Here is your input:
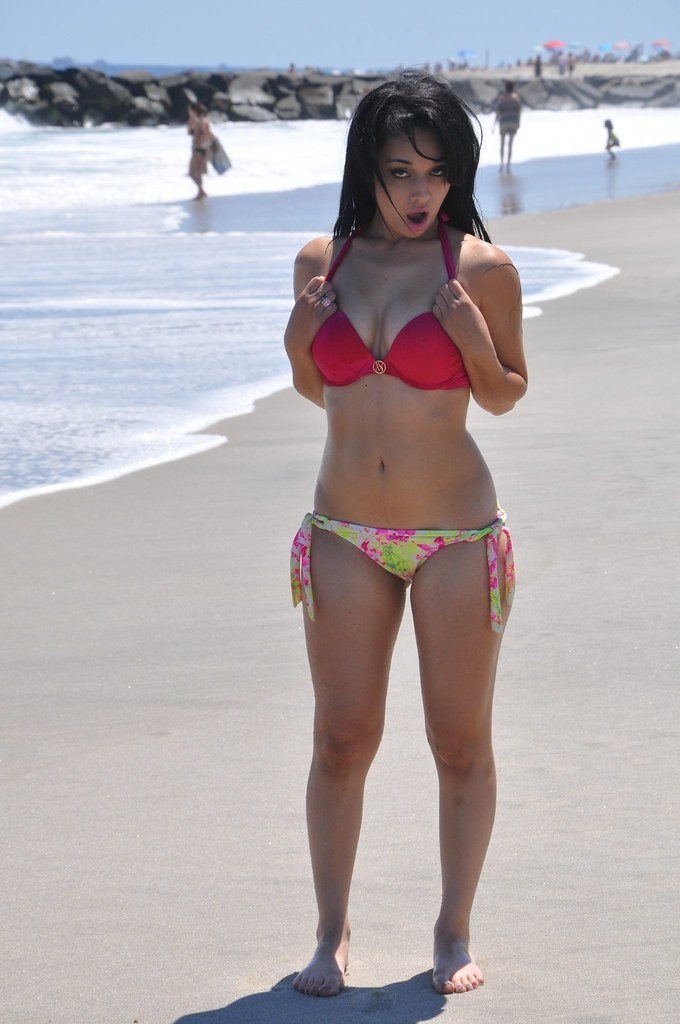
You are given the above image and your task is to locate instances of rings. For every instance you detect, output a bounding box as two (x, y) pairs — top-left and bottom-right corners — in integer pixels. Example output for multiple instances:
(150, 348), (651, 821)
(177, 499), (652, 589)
(320, 295), (332, 308)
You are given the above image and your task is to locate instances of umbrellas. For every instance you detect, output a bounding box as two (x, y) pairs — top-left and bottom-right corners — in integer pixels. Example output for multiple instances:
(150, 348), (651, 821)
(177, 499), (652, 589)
(459, 37), (672, 58)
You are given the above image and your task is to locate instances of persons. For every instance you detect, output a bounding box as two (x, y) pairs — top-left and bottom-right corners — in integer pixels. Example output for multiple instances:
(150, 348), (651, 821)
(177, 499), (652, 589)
(186, 102), (217, 200)
(281, 75), (530, 997)
(419, 49), (679, 76)
(604, 118), (621, 161)
(493, 80), (523, 171)
